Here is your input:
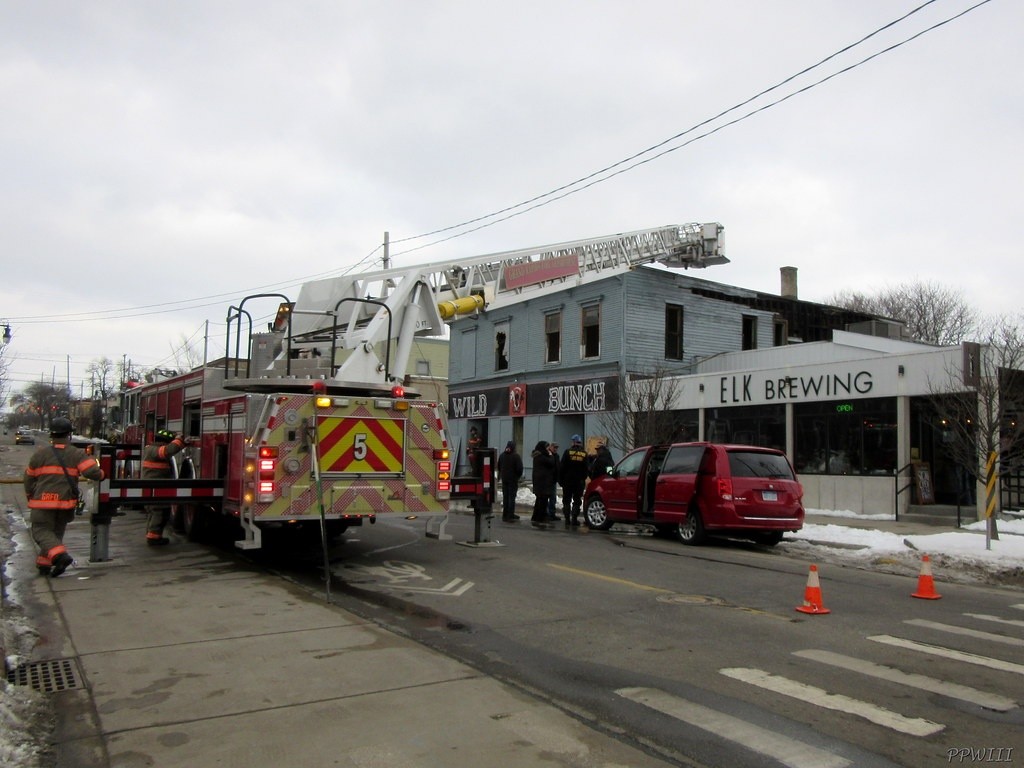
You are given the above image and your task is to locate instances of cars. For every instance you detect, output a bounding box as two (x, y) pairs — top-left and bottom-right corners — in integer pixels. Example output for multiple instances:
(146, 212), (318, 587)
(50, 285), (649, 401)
(15, 429), (35, 445)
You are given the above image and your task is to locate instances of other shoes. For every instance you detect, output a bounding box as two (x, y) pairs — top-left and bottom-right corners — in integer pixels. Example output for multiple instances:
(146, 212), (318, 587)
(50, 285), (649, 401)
(147, 537), (170, 546)
(51, 552), (73, 577)
(548, 514), (561, 521)
(502, 518), (515, 523)
(531, 517), (547, 522)
(512, 515), (520, 519)
(38, 565), (51, 575)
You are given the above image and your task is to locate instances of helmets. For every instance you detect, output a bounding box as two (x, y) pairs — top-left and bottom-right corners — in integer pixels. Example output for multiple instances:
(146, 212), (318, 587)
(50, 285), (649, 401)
(572, 434), (582, 443)
(50, 417), (77, 435)
(156, 430), (175, 442)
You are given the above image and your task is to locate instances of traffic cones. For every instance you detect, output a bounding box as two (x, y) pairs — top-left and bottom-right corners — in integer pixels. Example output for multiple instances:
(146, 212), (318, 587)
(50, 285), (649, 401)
(794, 561), (832, 614)
(910, 554), (942, 599)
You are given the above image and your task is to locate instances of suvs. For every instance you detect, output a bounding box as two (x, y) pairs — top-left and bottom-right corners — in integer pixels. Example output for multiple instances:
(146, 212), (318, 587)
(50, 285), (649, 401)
(583, 441), (805, 548)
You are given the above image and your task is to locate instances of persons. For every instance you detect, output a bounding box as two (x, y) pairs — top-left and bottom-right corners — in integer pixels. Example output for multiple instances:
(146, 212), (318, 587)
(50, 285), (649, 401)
(141, 429), (186, 546)
(109, 422), (123, 444)
(530, 440), (562, 528)
(24, 416), (106, 577)
(560, 435), (590, 527)
(589, 446), (615, 480)
(467, 426), (482, 476)
(497, 440), (523, 523)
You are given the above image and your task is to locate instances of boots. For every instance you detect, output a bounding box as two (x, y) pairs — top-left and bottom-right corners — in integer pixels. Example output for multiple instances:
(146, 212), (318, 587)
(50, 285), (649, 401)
(565, 514), (571, 525)
(571, 514), (580, 526)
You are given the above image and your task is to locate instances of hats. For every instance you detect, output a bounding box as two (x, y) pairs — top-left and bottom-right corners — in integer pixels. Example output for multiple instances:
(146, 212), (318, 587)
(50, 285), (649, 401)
(551, 442), (559, 447)
(470, 426), (478, 432)
(595, 444), (609, 451)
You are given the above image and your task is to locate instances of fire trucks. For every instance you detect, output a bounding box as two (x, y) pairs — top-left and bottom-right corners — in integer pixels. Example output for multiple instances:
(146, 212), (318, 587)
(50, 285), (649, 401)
(70, 220), (730, 571)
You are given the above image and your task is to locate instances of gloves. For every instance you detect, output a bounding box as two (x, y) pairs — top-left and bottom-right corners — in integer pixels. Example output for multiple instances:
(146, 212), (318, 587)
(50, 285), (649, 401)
(100, 468), (106, 482)
(176, 434), (185, 442)
